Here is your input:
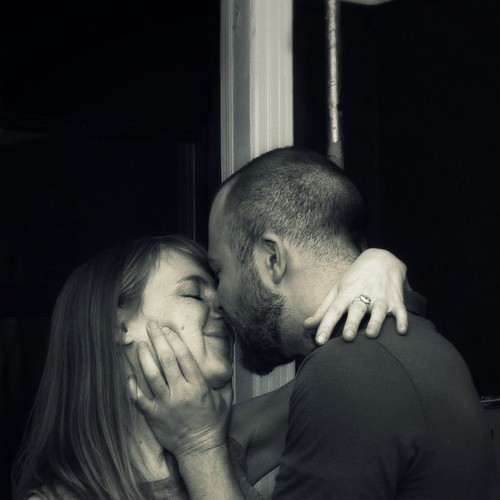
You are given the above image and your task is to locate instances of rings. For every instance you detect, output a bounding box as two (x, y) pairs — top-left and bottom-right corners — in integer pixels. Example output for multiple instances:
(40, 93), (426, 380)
(352, 293), (371, 311)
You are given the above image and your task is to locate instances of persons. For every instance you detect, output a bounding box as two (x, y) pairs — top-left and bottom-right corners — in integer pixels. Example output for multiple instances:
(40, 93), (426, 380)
(128, 146), (500, 500)
(11, 233), (412, 500)
